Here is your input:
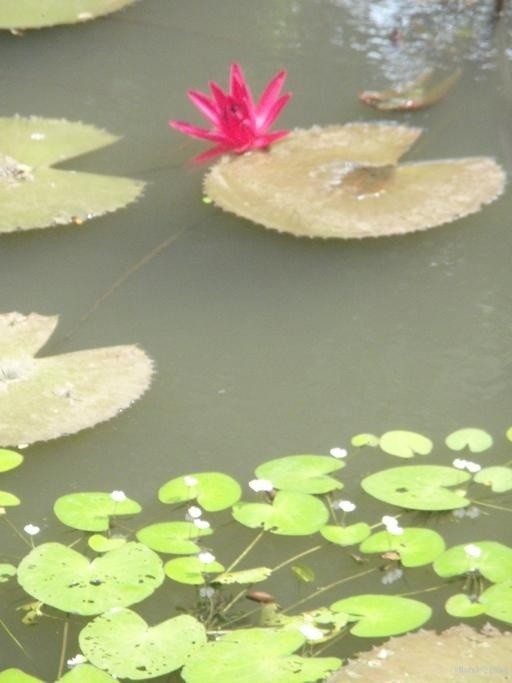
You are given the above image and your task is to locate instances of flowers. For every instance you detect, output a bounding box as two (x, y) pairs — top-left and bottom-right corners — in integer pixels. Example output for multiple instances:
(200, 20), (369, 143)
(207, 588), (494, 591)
(168, 64), (293, 166)
